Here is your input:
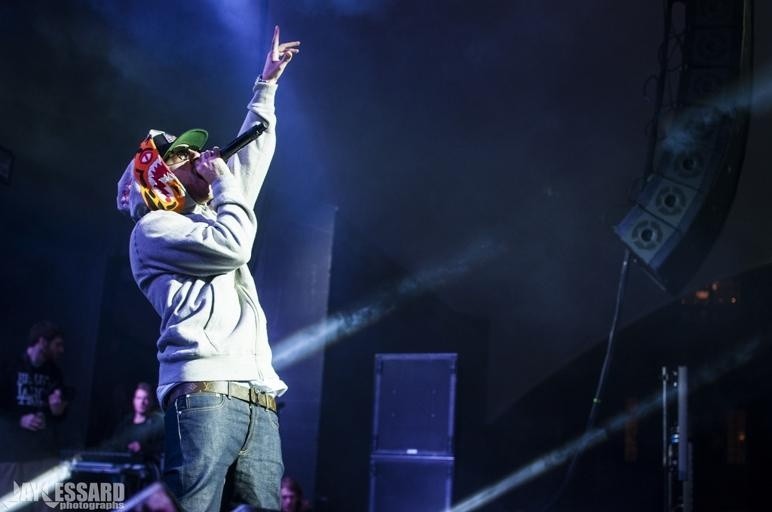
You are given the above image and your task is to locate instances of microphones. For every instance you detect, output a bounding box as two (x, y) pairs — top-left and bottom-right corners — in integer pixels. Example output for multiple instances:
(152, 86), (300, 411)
(192, 121), (269, 165)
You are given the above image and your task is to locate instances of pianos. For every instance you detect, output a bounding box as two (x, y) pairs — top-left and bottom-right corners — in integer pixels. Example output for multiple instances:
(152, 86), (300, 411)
(70, 451), (139, 465)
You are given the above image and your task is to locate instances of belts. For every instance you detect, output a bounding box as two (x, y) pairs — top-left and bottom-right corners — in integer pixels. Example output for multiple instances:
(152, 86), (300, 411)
(163, 381), (277, 413)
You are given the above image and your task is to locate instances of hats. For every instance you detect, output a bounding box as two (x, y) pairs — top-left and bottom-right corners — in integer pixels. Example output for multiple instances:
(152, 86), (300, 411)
(154, 128), (208, 163)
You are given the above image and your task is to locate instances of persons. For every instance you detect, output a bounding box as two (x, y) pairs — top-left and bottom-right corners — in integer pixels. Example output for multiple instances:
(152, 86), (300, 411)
(0, 318), (71, 462)
(110, 23), (303, 512)
(111, 380), (163, 492)
(281, 477), (311, 512)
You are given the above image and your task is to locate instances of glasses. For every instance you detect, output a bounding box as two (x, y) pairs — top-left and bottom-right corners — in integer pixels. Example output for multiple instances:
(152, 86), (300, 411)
(174, 146), (200, 160)
